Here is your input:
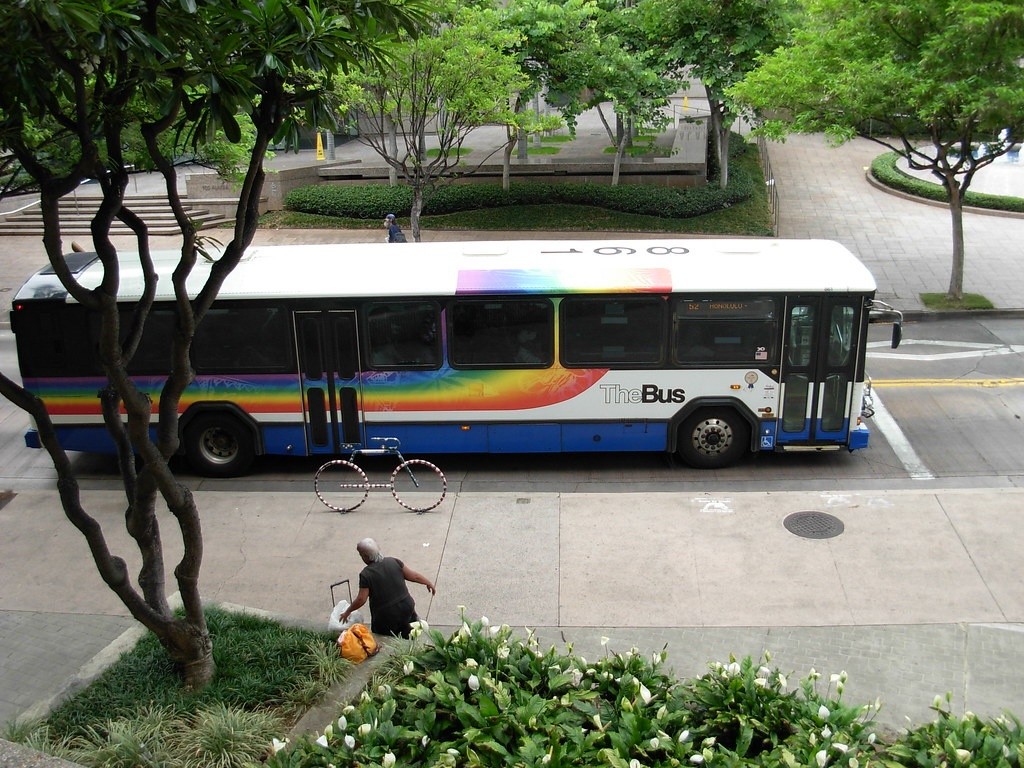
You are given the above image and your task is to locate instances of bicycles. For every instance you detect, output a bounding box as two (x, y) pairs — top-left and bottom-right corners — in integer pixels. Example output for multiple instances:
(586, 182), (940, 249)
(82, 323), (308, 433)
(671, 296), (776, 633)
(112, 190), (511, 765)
(313, 436), (448, 515)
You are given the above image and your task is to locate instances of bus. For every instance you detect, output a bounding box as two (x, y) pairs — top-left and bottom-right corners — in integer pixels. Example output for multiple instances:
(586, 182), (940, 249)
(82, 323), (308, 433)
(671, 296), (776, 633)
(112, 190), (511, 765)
(8, 238), (905, 479)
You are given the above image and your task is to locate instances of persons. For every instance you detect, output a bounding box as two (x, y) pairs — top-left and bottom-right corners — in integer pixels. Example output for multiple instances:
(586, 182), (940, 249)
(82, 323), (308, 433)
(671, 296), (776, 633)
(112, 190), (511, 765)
(339, 538), (436, 640)
(384, 214), (408, 243)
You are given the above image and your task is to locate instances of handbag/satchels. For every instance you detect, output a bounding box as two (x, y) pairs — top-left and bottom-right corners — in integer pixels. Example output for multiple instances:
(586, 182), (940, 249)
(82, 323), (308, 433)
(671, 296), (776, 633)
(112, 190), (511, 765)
(341, 623), (381, 663)
(329, 600), (364, 632)
